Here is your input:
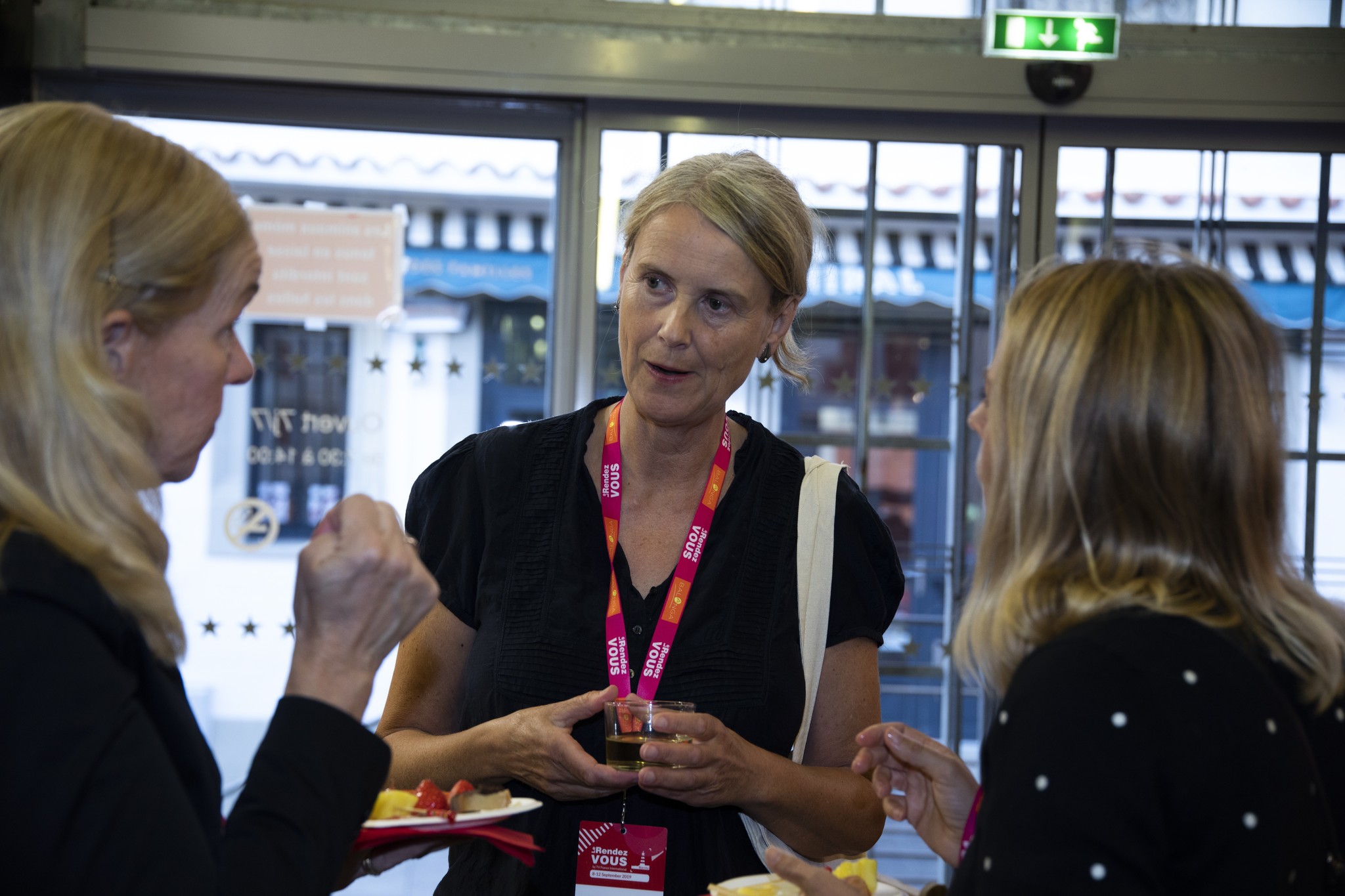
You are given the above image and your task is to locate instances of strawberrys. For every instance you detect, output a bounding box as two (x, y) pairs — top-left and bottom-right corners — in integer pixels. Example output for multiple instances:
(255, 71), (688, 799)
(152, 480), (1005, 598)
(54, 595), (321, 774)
(446, 779), (474, 805)
(412, 780), (449, 816)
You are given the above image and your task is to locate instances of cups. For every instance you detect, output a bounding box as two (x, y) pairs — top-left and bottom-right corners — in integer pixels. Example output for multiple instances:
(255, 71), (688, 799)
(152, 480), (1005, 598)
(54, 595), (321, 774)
(602, 697), (694, 772)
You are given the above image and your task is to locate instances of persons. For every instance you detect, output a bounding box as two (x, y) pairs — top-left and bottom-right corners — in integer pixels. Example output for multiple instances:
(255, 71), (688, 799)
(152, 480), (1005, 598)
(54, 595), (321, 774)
(375, 149), (905, 896)
(1, 102), (441, 896)
(762, 240), (1345, 896)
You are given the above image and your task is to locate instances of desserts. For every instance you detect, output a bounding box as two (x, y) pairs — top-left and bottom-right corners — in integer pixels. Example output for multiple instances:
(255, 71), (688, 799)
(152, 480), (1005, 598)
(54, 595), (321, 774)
(707, 857), (878, 896)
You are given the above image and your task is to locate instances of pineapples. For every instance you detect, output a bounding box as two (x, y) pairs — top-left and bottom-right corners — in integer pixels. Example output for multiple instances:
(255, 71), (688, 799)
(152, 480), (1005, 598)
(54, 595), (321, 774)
(369, 789), (418, 819)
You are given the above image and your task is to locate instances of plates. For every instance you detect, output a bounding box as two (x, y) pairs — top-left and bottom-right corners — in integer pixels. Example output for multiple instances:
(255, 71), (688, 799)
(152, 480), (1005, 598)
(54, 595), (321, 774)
(361, 796), (542, 828)
(709, 873), (905, 896)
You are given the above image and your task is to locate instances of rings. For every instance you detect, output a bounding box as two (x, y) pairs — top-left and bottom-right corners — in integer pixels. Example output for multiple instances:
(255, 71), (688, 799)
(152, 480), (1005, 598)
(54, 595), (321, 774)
(404, 535), (418, 550)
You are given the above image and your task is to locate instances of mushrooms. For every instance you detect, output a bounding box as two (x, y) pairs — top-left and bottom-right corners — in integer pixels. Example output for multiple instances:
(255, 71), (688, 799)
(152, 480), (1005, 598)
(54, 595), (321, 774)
(451, 789), (511, 812)
